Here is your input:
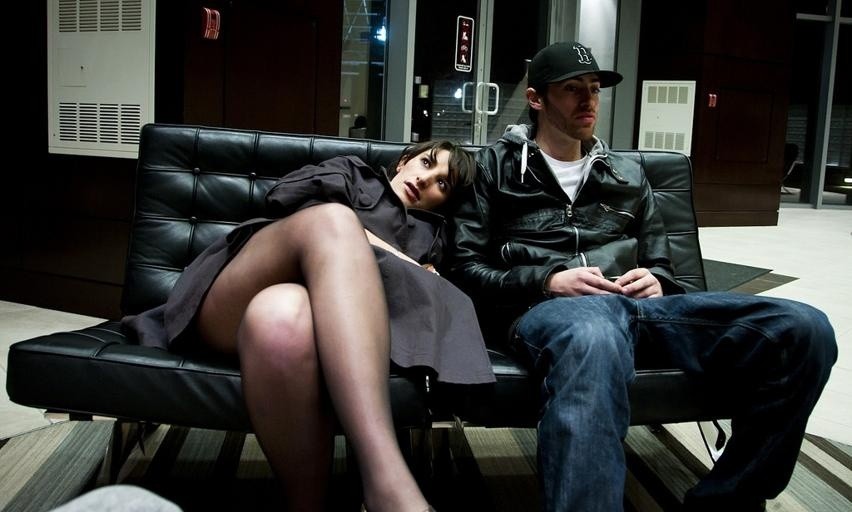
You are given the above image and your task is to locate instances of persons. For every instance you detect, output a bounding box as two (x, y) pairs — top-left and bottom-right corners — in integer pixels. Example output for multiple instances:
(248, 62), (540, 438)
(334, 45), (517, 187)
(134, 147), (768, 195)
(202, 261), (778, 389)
(454, 42), (838, 512)
(122, 138), (498, 511)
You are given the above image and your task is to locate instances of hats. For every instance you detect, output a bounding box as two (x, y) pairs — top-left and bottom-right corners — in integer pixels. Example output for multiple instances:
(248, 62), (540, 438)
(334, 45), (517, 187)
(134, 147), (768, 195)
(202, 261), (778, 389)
(528, 41), (623, 88)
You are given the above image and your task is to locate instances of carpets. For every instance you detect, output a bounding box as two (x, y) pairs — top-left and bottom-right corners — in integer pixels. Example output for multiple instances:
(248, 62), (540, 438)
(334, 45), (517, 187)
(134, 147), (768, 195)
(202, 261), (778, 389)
(1, 421), (852, 512)
(702, 259), (801, 295)
